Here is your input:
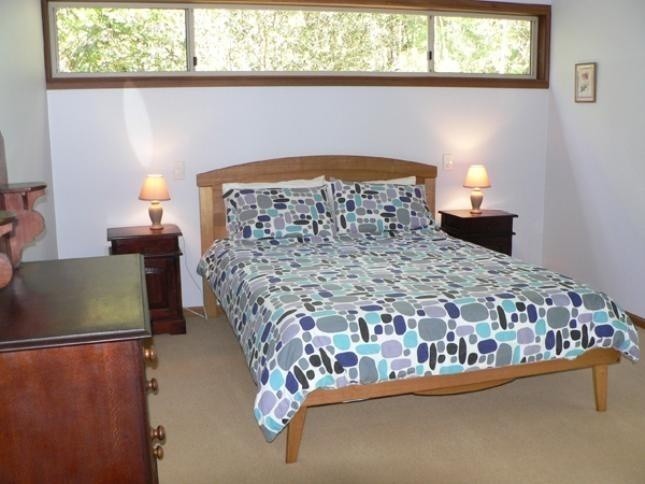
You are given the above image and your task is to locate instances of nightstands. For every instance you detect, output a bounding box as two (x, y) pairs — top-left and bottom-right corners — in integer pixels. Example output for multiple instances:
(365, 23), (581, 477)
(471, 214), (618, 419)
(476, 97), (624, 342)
(438, 208), (519, 258)
(104, 222), (191, 337)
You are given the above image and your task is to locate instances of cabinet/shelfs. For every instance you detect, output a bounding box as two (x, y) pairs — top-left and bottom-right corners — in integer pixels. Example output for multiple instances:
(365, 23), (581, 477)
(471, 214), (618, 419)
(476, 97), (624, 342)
(0, 250), (166, 483)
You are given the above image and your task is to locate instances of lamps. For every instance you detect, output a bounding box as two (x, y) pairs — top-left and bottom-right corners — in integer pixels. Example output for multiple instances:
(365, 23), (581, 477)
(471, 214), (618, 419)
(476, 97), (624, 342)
(135, 171), (174, 230)
(463, 162), (491, 215)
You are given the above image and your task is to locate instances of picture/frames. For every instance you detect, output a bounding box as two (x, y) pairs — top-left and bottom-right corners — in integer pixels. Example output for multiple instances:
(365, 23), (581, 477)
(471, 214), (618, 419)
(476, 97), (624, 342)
(574, 59), (597, 103)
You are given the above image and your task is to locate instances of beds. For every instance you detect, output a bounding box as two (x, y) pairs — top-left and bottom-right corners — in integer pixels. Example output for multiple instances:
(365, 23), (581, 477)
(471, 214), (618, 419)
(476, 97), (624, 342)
(193, 150), (641, 465)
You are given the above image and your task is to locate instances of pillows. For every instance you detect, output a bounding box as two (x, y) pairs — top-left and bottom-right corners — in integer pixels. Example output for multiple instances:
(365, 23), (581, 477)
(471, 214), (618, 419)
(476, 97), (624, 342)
(329, 173), (417, 185)
(219, 170), (327, 191)
(332, 178), (436, 234)
(219, 184), (336, 246)
